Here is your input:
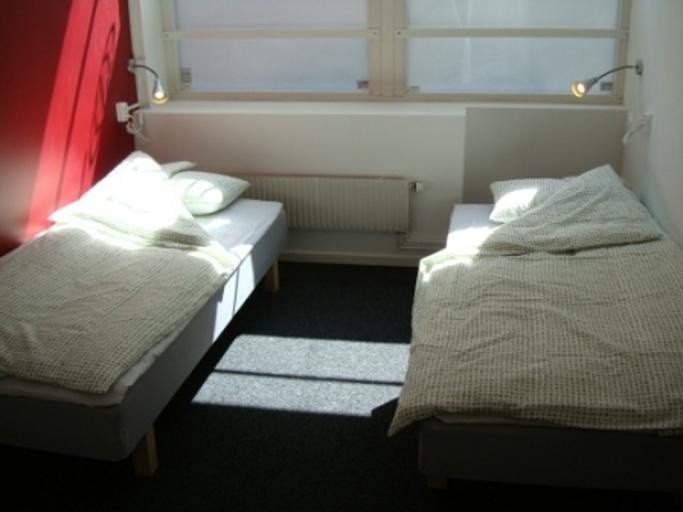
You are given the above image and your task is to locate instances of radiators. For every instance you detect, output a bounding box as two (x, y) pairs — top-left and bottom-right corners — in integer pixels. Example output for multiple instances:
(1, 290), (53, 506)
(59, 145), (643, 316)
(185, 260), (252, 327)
(231, 170), (411, 236)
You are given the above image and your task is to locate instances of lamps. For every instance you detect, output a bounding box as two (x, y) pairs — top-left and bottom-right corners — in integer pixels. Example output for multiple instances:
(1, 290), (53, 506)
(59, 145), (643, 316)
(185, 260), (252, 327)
(128, 59), (169, 105)
(570, 59), (643, 99)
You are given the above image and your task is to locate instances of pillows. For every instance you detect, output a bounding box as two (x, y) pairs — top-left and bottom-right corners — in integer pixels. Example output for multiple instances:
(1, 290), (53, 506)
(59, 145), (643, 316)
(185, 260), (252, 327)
(154, 157), (253, 216)
(487, 176), (581, 224)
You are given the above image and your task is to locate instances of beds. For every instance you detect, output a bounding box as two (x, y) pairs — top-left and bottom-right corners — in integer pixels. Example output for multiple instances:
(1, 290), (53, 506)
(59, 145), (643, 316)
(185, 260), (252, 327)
(417, 199), (680, 499)
(2, 191), (295, 481)
